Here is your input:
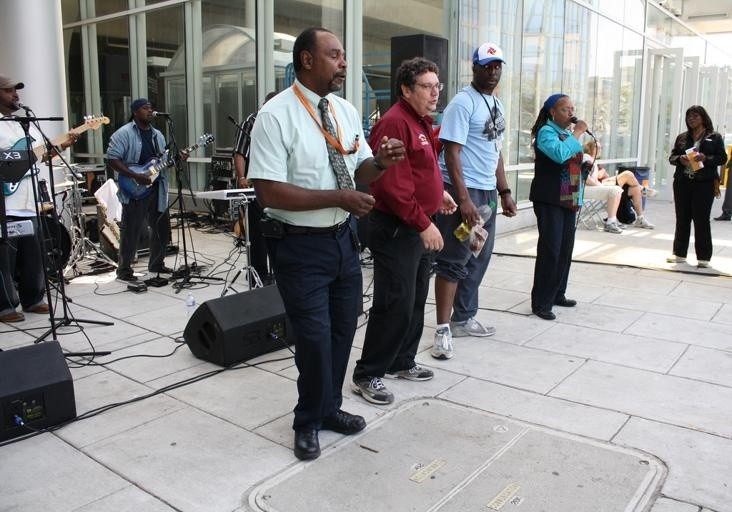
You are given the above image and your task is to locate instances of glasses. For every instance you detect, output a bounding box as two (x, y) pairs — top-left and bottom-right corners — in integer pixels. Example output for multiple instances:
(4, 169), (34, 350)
(416, 82), (444, 91)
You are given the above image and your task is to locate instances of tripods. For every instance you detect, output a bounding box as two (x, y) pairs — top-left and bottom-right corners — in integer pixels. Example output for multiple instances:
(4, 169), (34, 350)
(0, 116), (115, 358)
(164, 124), (224, 295)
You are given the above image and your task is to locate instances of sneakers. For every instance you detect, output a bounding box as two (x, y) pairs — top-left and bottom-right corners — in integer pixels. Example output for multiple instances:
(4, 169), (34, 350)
(714, 214), (731, 220)
(450, 317), (496, 338)
(697, 260), (708, 267)
(634, 218), (654, 229)
(640, 186), (657, 198)
(350, 377), (394, 404)
(603, 222), (622, 234)
(667, 255), (685, 263)
(432, 327), (453, 360)
(384, 364), (433, 381)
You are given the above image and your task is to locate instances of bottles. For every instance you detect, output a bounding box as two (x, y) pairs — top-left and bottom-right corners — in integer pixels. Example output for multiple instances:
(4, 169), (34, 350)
(454, 201), (496, 242)
(186, 291), (197, 322)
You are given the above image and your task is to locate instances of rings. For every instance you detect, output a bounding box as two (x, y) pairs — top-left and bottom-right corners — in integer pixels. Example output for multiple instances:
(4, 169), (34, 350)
(74, 139), (78, 142)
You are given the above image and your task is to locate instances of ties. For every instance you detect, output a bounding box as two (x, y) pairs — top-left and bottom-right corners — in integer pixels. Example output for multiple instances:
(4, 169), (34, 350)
(318, 98), (354, 189)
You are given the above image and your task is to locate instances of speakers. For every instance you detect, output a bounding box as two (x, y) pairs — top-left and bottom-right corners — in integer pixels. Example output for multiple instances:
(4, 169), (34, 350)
(0, 339), (77, 447)
(182, 283), (296, 368)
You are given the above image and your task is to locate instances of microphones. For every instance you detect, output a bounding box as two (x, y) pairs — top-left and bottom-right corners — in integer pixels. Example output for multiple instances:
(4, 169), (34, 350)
(570, 115), (592, 137)
(151, 109), (171, 121)
(226, 113), (250, 137)
(13, 101), (33, 112)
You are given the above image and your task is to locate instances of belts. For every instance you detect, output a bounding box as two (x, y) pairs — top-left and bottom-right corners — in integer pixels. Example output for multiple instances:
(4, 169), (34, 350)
(282, 220), (348, 233)
(678, 173), (702, 180)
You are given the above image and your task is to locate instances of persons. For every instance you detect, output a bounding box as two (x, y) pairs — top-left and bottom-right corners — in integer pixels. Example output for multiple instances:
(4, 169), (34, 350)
(104, 97), (192, 281)
(712, 154), (732, 222)
(429, 35), (519, 364)
(527, 93), (601, 322)
(582, 139), (627, 235)
(664, 105), (728, 269)
(245, 25), (409, 462)
(346, 52), (460, 407)
(0, 73), (82, 322)
(232, 89), (277, 289)
(581, 163), (653, 234)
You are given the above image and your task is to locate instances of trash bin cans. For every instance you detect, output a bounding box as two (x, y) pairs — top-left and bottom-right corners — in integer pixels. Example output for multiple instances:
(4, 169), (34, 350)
(617, 166), (651, 211)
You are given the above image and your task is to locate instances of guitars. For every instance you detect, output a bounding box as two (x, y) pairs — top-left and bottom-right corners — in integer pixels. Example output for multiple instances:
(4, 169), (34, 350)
(3, 113), (110, 197)
(119, 134), (215, 201)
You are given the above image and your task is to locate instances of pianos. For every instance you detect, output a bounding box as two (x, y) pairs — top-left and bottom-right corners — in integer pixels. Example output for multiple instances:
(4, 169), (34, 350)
(196, 185), (255, 200)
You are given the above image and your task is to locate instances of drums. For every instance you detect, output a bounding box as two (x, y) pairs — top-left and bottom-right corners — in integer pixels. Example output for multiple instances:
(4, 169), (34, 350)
(37, 200), (74, 270)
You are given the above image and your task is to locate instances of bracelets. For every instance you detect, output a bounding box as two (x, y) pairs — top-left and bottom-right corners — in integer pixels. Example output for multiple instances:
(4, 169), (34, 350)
(373, 156), (387, 172)
(56, 144), (63, 152)
(239, 176), (245, 180)
(497, 187), (511, 196)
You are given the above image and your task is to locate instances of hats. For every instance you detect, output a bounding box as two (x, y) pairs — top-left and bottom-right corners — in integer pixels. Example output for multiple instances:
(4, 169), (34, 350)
(128, 98), (151, 122)
(473, 43), (506, 66)
(0, 74), (24, 89)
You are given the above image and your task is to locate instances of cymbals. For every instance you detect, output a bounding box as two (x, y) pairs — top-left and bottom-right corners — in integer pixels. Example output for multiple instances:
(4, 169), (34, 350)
(53, 180), (85, 187)
(46, 163), (77, 168)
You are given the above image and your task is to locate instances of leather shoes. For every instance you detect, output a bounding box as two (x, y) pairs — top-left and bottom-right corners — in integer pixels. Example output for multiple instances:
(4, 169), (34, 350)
(0, 313), (25, 322)
(149, 266), (173, 273)
(532, 310), (556, 320)
(555, 300), (576, 306)
(116, 274), (137, 281)
(321, 409), (366, 434)
(294, 429), (321, 460)
(23, 304), (49, 313)
